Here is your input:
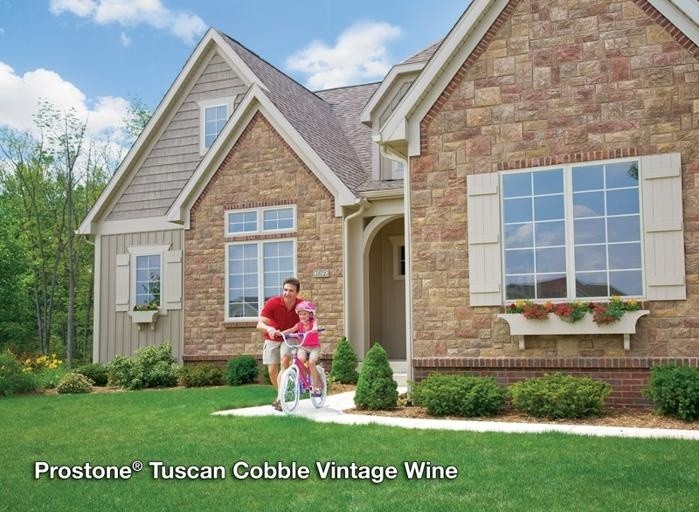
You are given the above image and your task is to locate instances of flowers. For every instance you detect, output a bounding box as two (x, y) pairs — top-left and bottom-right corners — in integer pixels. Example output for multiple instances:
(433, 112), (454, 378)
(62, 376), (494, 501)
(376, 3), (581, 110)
(507, 295), (640, 326)
(133, 303), (158, 311)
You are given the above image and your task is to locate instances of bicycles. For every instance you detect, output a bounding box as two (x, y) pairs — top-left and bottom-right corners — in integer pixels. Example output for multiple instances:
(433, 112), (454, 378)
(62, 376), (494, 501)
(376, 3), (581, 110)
(265, 327), (330, 415)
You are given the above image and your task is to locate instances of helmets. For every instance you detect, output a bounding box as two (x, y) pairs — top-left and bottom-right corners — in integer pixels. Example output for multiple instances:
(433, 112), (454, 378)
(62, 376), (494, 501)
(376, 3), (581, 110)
(295, 301), (316, 316)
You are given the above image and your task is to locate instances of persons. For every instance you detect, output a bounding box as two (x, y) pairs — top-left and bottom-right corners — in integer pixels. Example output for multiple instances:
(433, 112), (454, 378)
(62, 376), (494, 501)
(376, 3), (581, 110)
(275, 301), (322, 393)
(256, 278), (304, 412)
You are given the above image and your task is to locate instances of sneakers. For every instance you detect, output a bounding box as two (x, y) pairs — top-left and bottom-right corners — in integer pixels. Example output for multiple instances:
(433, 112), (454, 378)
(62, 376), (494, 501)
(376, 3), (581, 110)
(311, 382), (325, 397)
(272, 397), (283, 411)
